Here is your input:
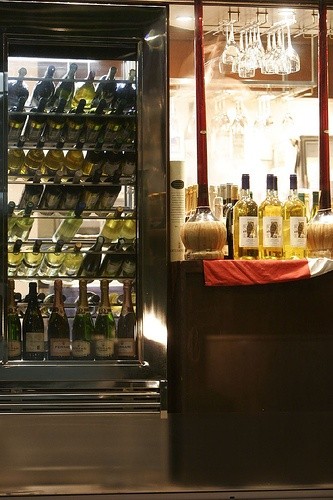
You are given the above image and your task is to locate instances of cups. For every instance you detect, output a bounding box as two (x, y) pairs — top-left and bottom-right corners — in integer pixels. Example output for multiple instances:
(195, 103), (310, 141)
(169, 159), (187, 262)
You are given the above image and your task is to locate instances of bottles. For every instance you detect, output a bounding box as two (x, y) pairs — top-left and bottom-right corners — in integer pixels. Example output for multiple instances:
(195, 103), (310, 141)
(311, 192), (319, 219)
(186, 171), (286, 257)
(5, 63), (138, 361)
(285, 174), (307, 256)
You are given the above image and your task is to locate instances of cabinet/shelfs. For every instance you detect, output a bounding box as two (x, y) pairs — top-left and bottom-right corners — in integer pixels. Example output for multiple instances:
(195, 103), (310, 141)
(2, 1), (168, 381)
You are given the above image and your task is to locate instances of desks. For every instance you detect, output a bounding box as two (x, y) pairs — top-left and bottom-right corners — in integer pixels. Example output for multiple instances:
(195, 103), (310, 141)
(168, 256), (333, 500)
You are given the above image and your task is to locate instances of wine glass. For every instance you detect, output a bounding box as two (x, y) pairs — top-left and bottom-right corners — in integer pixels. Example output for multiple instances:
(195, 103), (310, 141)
(220, 19), (301, 78)
(213, 84), (298, 139)
